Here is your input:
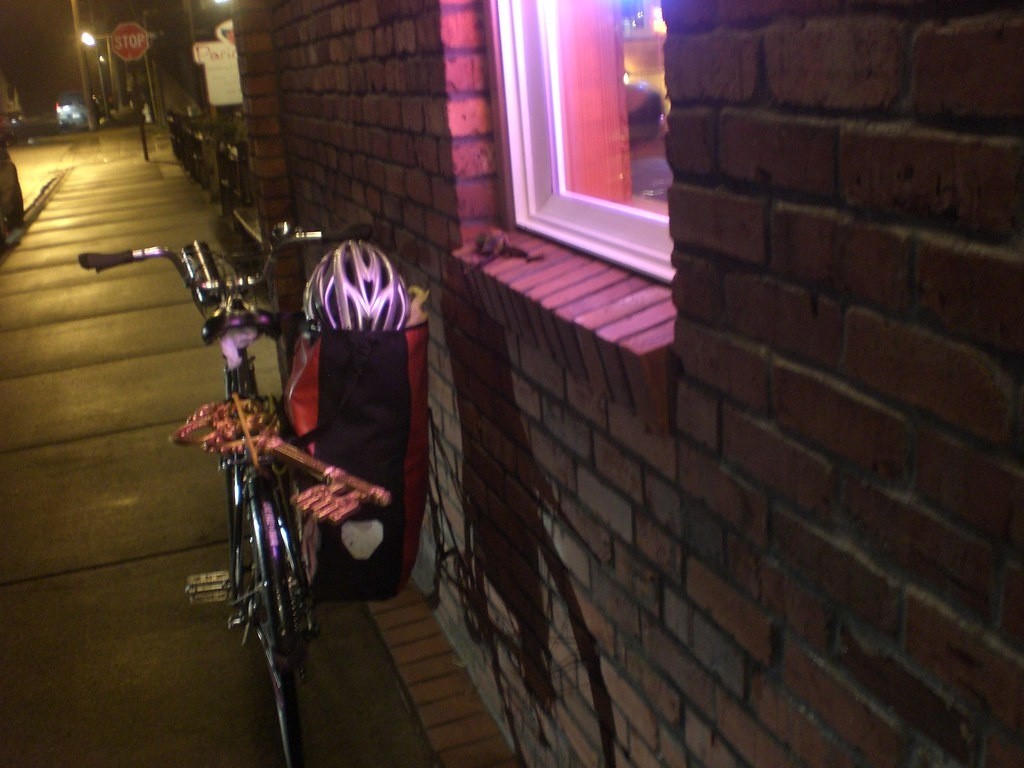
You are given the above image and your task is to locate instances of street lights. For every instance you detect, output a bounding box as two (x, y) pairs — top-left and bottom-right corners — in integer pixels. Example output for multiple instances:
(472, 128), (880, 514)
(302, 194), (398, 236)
(81, 31), (125, 117)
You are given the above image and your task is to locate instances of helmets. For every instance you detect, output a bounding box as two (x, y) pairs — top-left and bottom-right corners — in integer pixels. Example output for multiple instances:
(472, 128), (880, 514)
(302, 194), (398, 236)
(302, 239), (409, 332)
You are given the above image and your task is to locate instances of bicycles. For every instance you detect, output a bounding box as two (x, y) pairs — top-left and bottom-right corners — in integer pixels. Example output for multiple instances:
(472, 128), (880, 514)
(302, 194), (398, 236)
(76, 219), (376, 768)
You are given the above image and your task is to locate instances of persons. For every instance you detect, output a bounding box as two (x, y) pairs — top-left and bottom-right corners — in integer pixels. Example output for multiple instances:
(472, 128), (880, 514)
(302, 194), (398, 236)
(0, 138), (24, 242)
(625, 83), (674, 202)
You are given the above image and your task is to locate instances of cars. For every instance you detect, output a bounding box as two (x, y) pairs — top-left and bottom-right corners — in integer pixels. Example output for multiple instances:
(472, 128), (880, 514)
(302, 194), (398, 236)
(55, 92), (100, 130)
(0, 139), (25, 244)
(0, 109), (27, 146)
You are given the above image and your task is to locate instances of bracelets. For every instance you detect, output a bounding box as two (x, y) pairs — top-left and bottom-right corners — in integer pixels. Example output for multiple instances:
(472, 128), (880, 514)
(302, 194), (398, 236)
(3, 216), (8, 221)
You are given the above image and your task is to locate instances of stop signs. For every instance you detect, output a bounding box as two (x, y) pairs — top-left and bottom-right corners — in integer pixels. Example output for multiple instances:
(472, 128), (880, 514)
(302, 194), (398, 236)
(111, 21), (150, 61)
